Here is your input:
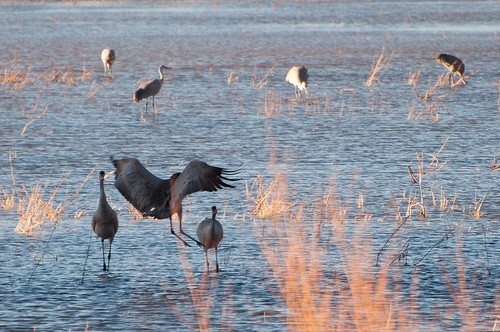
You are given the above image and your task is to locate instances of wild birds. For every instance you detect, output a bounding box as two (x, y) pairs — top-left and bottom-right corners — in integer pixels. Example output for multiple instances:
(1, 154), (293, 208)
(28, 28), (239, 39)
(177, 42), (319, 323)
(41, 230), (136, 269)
(434, 54), (467, 85)
(101, 48), (116, 73)
(133, 64), (172, 113)
(111, 158), (248, 248)
(285, 64), (309, 95)
(91, 171), (118, 271)
(197, 206), (223, 274)
